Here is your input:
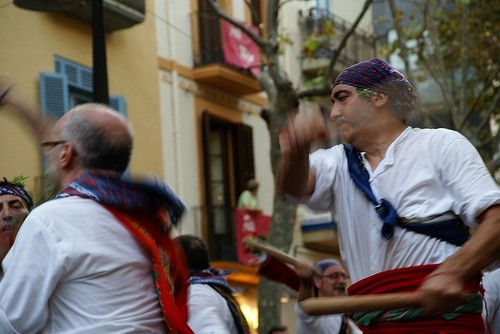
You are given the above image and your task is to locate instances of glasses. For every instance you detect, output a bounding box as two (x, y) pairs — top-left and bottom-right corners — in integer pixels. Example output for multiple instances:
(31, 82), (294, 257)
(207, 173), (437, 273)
(319, 272), (348, 280)
(41, 140), (79, 158)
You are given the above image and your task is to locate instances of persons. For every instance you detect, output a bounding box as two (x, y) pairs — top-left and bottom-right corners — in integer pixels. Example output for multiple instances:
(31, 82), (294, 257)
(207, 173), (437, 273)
(236, 179), (262, 210)
(0, 178), (34, 279)
(173, 235), (250, 334)
(481, 267), (500, 334)
(0, 102), (195, 334)
(276, 59), (500, 333)
(268, 258), (363, 334)
